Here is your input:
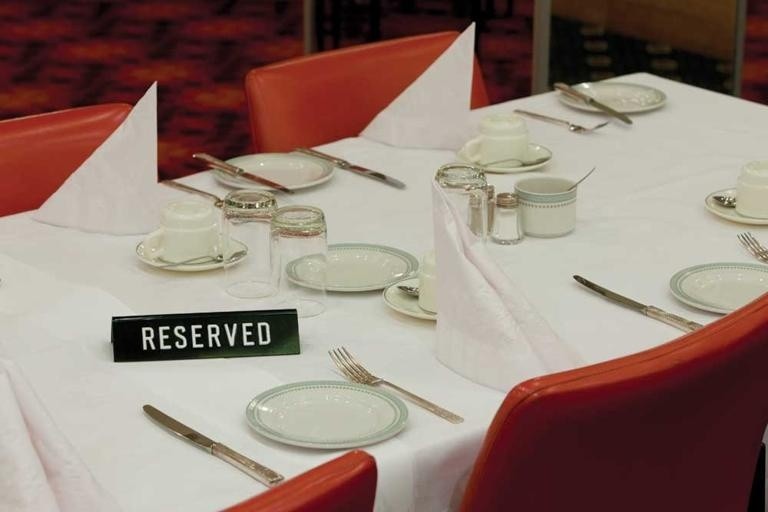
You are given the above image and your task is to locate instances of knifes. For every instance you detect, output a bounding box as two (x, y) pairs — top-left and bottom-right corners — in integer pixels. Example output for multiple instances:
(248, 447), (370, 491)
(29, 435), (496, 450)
(138, 401), (290, 488)
(568, 273), (706, 334)
(394, 279), (422, 300)
(294, 143), (410, 191)
(552, 80), (633, 126)
(194, 148), (296, 199)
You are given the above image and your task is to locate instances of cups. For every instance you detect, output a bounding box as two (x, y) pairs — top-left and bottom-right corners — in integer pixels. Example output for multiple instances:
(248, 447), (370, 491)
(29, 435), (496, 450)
(142, 200), (223, 260)
(514, 175), (576, 238)
(436, 163), (487, 249)
(477, 113), (531, 166)
(417, 251), (439, 313)
(733, 160), (768, 218)
(265, 206), (333, 320)
(220, 190), (282, 297)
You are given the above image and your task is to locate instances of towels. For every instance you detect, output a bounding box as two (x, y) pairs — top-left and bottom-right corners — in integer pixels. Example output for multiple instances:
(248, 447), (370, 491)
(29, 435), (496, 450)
(426, 176), (590, 390)
(31, 77), (168, 236)
(356, 20), (481, 154)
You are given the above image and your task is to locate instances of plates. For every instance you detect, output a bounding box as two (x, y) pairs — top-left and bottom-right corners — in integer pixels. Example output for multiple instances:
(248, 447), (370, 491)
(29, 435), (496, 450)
(458, 135), (555, 171)
(382, 279), (438, 324)
(667, 262), (768, 314)
(210, 152), (332, 191)
(288, 241), (420, 294)
(245, 378), (407, 452)
(705, 186), (768, 225)
(560, 81), (667, 115)
(135, 230), (248, 272)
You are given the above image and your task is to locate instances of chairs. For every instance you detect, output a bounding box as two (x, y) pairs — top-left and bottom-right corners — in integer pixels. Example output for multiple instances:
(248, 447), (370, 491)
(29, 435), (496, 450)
(223, 449), (380, 512)
(455, 289), (766, 509)
(0, 103), (138, 221)
(241, 29), (491, 152)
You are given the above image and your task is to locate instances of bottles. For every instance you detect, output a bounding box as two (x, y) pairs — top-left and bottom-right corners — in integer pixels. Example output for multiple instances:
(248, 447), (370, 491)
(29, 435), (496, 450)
(471, 180), (522, 247)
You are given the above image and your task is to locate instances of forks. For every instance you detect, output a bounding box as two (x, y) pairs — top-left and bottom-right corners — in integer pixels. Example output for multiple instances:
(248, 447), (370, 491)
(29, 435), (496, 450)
(514, 106), (609, 134)
(737, 230), (768, 266)
(328, 345), (468, 427)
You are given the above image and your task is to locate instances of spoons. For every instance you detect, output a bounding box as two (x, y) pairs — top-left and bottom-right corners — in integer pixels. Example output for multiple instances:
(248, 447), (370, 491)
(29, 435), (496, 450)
(712, 192), (735, 209)
